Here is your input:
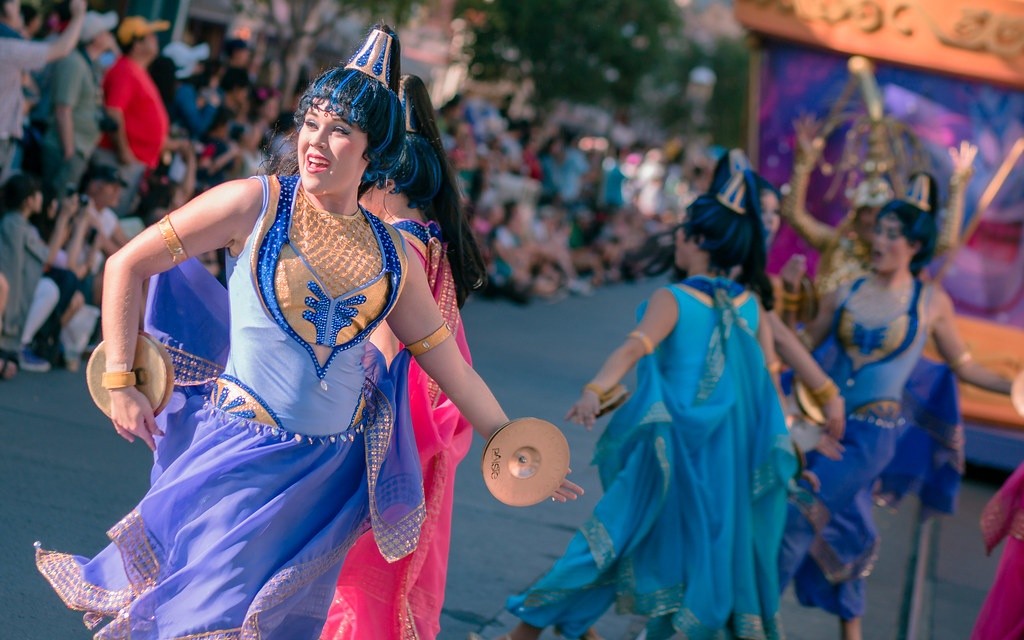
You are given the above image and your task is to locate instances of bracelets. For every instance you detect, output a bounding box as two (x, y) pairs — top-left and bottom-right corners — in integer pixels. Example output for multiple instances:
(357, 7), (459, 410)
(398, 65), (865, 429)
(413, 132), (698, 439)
(470, 190), (800, 640)
(582, 384), (605, 401)
(101, 372), (137, 390)
(812, 379), (838, 405)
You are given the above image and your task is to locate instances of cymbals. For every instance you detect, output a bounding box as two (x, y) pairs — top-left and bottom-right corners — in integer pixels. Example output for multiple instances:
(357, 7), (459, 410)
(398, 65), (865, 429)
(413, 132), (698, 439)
(481, 415), (575, 507)
(85, 328), (176, 423)
(788, 372), (837, 428)
(574, 382), (633, 422)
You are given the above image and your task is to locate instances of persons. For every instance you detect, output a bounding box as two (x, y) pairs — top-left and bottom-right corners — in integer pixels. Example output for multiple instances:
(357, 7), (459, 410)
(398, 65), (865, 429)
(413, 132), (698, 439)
(97, 64), (586, 639)
(430, 91), (729, 307)
(778, 199), (1016, 640)
(320, 70), (493, 640)
(785, 111), (978, 311)
(0, 1), (333, 381)
(972, 366), (1024, 640)
(747, 172), (849, 440)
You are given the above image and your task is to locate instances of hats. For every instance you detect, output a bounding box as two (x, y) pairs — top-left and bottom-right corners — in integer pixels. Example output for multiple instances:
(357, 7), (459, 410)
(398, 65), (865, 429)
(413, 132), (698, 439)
(76, 9), (117, 44)
(79, 164), (131, 193)
(161, 41), (211, 79)
(1, 173), (42, 210)
(117, 16), (170, 42)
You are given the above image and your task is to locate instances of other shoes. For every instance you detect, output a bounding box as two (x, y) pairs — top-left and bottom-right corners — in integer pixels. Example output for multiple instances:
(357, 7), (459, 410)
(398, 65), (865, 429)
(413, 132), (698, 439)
(18, 343), (50, 372)
